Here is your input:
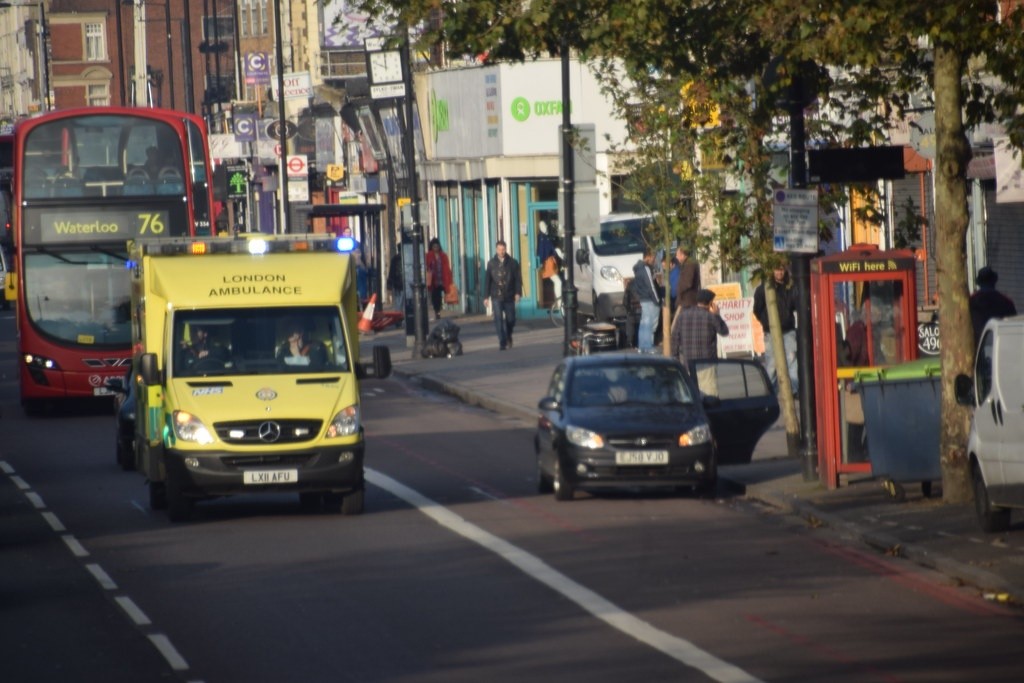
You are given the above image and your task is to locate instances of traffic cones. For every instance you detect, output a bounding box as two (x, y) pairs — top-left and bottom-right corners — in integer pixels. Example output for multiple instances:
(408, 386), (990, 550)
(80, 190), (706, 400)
(357, 293), (377, 335)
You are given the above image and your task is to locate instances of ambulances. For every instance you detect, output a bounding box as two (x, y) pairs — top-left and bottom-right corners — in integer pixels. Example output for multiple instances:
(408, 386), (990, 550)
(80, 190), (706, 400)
(124, 233), (394, 522)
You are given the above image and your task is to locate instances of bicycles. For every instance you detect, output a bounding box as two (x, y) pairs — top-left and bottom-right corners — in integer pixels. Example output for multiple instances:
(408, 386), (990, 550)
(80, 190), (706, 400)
(550, 295), (565, 329)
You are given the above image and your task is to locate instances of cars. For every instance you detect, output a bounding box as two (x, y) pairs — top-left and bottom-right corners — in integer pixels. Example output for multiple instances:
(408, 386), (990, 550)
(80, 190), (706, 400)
(533, 355), (781, 501)
(103, 365), (139, 470)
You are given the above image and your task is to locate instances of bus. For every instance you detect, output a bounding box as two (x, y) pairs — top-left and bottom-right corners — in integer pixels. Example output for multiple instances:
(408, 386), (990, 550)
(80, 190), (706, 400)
(0, 108), (216, 418)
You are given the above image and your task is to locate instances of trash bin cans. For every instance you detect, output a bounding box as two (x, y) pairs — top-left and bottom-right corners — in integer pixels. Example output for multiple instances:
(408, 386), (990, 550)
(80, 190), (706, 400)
(578, 324), (618, 357)
(851, 356), (941, 498)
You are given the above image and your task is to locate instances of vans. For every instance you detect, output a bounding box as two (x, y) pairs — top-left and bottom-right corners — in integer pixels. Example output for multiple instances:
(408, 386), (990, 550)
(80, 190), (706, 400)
(571, 211), (679, 349)
(952, 313), (1024, 533)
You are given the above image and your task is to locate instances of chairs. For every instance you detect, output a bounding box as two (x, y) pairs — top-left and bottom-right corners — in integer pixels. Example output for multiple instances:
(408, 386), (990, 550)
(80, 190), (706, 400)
(24, 167), (51, 198)
(156, 167), (185, 195)
(122, 167), (155, 196)
(573, 376), (607, 406)
(50, 167), (85, 197)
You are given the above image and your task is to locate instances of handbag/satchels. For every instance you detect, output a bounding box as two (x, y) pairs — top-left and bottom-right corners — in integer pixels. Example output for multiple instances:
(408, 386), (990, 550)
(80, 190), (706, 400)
(444, 283), (459, 305)
(425, 271), (433, 286)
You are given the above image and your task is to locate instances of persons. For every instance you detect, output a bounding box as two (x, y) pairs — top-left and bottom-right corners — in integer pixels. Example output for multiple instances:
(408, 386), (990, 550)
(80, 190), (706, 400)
(179, 325), (238, 376)
(623, 246), (902, 400)
(538, 221), (564, 311)
(328, 226), (523, 351)
(969, 267), (1016, 395)
(274, 315), (328, 367)
